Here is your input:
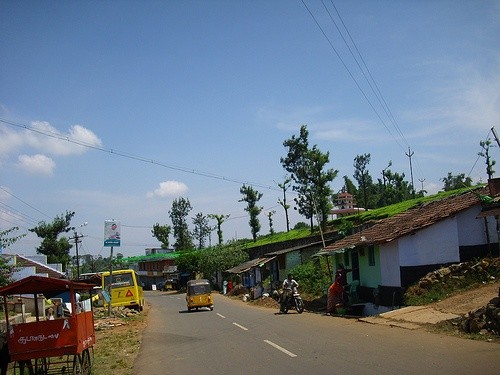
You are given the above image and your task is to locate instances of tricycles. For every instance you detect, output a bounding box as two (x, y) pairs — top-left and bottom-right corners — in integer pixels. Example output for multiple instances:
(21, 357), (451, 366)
(186, 279), (214, 311)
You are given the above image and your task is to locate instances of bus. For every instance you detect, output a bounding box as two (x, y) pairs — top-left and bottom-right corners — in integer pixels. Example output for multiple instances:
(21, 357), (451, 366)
(102, 270), (145, 312)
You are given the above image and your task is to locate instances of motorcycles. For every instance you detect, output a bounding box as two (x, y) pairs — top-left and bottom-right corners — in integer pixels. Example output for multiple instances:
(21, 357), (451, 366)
(279, 287), (305, 314)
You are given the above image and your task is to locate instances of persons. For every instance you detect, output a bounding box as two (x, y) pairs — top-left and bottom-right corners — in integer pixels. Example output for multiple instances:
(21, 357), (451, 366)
(105, 223), (120, 241)
(327, 274), (345, 316)
(279, 273), (299, 314)
(55, 300), (66, 317)
(76, 293), (80, 302)
(223, 278), (232, 296)
(480, 287), (500, 342)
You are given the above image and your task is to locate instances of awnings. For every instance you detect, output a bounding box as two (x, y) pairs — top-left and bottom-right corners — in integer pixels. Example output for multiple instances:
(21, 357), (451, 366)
(226, 257), (276, 276)
